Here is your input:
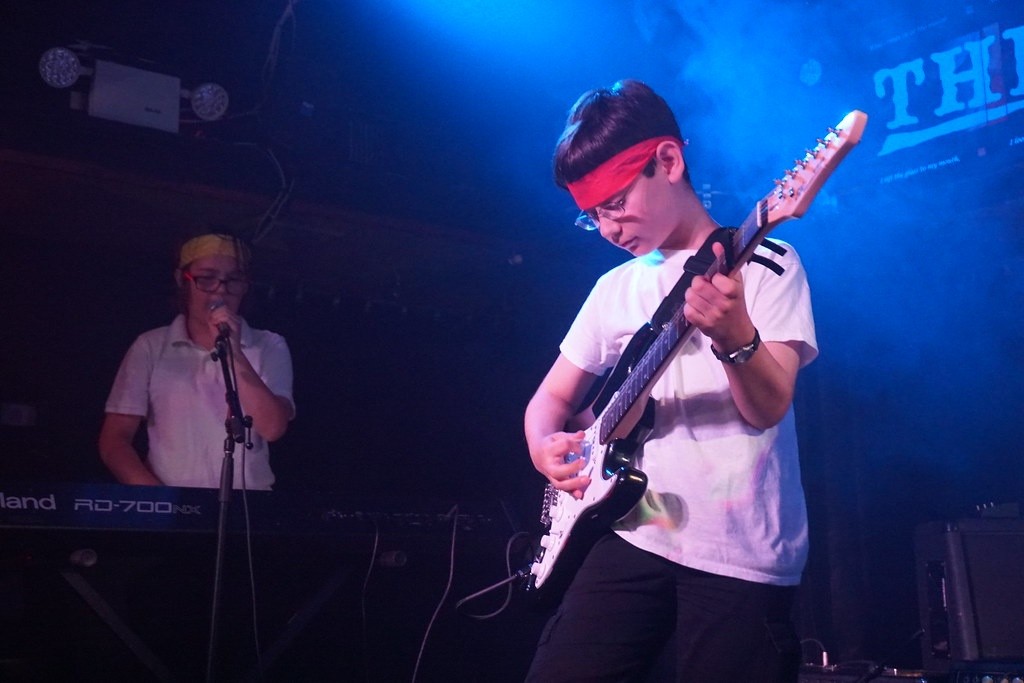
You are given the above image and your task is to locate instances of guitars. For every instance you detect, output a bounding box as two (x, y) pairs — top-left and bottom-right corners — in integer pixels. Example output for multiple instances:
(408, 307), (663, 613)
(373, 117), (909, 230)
(523, 107), (868, 608)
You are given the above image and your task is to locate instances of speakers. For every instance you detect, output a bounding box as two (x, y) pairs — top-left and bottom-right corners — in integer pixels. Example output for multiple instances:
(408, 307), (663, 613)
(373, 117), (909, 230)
(913, 516), (1024, 675)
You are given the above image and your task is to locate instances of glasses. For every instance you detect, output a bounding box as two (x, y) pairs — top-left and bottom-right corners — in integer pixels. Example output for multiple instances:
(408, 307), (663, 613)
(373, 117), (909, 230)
(182, 269), (250, 296)
(574, 181), (635, 232)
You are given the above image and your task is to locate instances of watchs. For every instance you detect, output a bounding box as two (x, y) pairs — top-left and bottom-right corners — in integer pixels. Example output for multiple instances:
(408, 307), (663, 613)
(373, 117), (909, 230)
(710, 327), (760, 365)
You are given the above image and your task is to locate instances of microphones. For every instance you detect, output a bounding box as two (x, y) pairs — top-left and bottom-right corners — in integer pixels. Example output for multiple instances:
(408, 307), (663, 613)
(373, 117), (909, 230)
(210, 303), (230, 338)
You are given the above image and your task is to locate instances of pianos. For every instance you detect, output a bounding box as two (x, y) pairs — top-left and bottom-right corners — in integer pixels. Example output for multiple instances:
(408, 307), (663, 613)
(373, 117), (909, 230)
(0, 477), (523, 547)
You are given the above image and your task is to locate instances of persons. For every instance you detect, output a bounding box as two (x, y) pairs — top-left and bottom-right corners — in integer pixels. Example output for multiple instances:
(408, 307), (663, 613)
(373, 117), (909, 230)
(99, 223), (297, 683)
(523, 78), (818, 683)
